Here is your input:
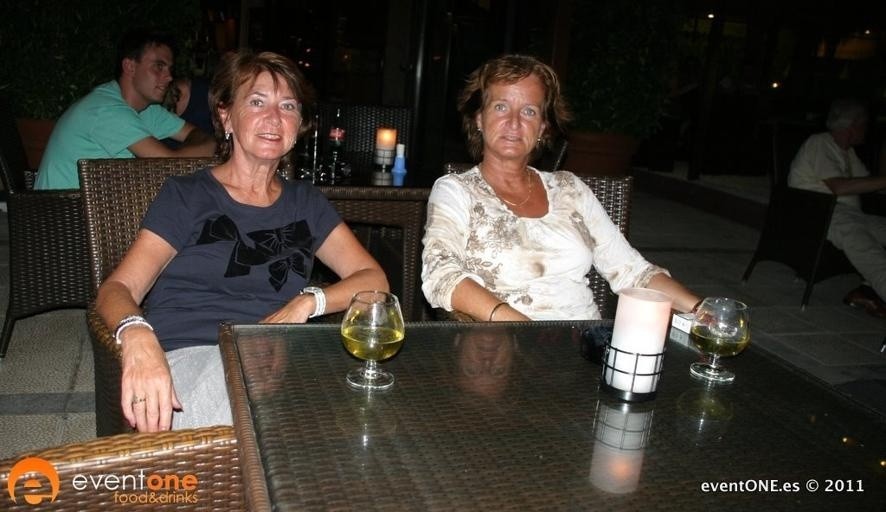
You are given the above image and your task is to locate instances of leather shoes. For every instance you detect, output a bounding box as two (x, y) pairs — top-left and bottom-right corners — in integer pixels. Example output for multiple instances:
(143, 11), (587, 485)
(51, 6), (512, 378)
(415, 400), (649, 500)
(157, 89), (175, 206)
(843, 283), (886, 318)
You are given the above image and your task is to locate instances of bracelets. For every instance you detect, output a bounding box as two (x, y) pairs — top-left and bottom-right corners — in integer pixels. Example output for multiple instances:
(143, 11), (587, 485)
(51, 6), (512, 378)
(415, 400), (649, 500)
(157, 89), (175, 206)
(690, 299), (704, 314)
(489, 302), (509, 322)
(112, 315), (154, 344)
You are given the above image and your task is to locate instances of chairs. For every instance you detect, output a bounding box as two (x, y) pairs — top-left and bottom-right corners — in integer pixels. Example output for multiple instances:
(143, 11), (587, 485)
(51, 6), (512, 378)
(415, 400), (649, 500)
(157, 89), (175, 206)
(0, 101), (886, 512)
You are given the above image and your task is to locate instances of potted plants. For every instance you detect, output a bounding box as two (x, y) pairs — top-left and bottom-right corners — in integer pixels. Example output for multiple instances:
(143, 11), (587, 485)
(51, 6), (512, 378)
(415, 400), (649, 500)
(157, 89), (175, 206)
(555, 0), (686, 179)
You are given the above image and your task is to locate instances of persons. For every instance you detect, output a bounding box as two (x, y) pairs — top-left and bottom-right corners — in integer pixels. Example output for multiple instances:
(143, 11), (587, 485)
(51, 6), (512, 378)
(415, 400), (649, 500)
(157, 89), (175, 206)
(168, 75), (191, 121)
(447, 321), (525, 416)
(94, 51), (391, 432)
(786, 97), (886, 321)
(32, 25), (222, 194)
(235, 333), (291, 406)
(422, 54), (716, 322)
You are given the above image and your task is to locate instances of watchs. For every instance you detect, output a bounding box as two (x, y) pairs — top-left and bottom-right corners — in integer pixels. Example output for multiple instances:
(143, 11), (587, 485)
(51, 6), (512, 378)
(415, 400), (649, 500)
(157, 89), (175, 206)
(299, 286), (327, 319)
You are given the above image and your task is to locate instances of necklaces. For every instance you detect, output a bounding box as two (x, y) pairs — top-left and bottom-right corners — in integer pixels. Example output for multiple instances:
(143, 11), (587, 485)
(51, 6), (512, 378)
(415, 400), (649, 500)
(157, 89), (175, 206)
(479, 162), (533, 207)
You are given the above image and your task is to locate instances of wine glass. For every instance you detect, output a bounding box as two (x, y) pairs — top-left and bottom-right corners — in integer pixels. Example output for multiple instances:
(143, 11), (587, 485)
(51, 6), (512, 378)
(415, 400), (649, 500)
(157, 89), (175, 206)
(334, 385), (399, 445)
(340, 290), (406, 391)
(677, 379), (736, 446)
(689, 295), (752, 383)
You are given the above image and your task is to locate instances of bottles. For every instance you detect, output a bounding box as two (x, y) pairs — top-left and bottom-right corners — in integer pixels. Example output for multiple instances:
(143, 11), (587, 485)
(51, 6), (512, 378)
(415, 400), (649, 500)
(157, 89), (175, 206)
(326, 108), (347, 155)
